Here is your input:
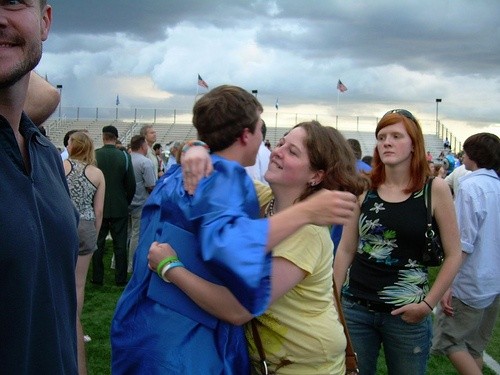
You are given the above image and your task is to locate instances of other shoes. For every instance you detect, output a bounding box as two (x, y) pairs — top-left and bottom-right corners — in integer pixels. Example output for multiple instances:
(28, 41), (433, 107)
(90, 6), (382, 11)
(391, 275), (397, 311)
(90, 278), (103, 285)
(127, 265), (133, 273)
(116, 281), (128, 285)
(105, 233), (113, 241)
(111, 262), (116, 269)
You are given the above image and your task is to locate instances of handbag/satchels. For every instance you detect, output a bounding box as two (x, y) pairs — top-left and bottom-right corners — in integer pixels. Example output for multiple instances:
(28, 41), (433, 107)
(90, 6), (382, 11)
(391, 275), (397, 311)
(122, 150), (136, 206)
(420, 177), (444, 267)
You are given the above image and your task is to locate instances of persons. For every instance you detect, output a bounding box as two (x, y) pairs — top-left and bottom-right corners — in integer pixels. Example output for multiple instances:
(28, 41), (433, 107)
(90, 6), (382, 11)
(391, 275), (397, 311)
(26, 0), (500, 375)
(0, 0), (81, 375)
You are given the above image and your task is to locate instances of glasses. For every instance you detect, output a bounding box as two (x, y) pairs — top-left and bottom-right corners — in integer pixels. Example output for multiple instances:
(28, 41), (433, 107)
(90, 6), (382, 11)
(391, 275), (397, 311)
(385, 109), (419, 127)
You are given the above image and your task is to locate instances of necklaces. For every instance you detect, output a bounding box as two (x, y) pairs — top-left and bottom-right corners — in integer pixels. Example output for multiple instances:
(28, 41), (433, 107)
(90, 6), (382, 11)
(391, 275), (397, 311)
(269, 197), (275, 216)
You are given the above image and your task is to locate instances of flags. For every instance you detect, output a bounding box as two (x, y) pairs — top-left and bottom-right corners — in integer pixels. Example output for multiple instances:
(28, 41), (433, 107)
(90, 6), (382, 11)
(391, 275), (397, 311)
(337, 81), (347, 92)
(198, 74), (208, 88)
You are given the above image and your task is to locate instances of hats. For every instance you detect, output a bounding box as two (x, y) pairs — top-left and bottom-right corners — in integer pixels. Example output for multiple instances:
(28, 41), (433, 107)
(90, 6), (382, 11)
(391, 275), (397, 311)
(103, 125), (118, 136)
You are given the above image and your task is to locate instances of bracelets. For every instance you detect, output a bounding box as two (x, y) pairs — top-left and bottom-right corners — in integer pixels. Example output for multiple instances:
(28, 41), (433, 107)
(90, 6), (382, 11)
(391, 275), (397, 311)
(181, 140), (210, 155)
(422, 299), (433, 311)
(157, 256), (184, 283)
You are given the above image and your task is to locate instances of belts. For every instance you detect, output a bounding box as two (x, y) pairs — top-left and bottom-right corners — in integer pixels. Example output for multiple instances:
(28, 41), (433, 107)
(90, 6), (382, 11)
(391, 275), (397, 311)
(342, 293), (401, 314)
